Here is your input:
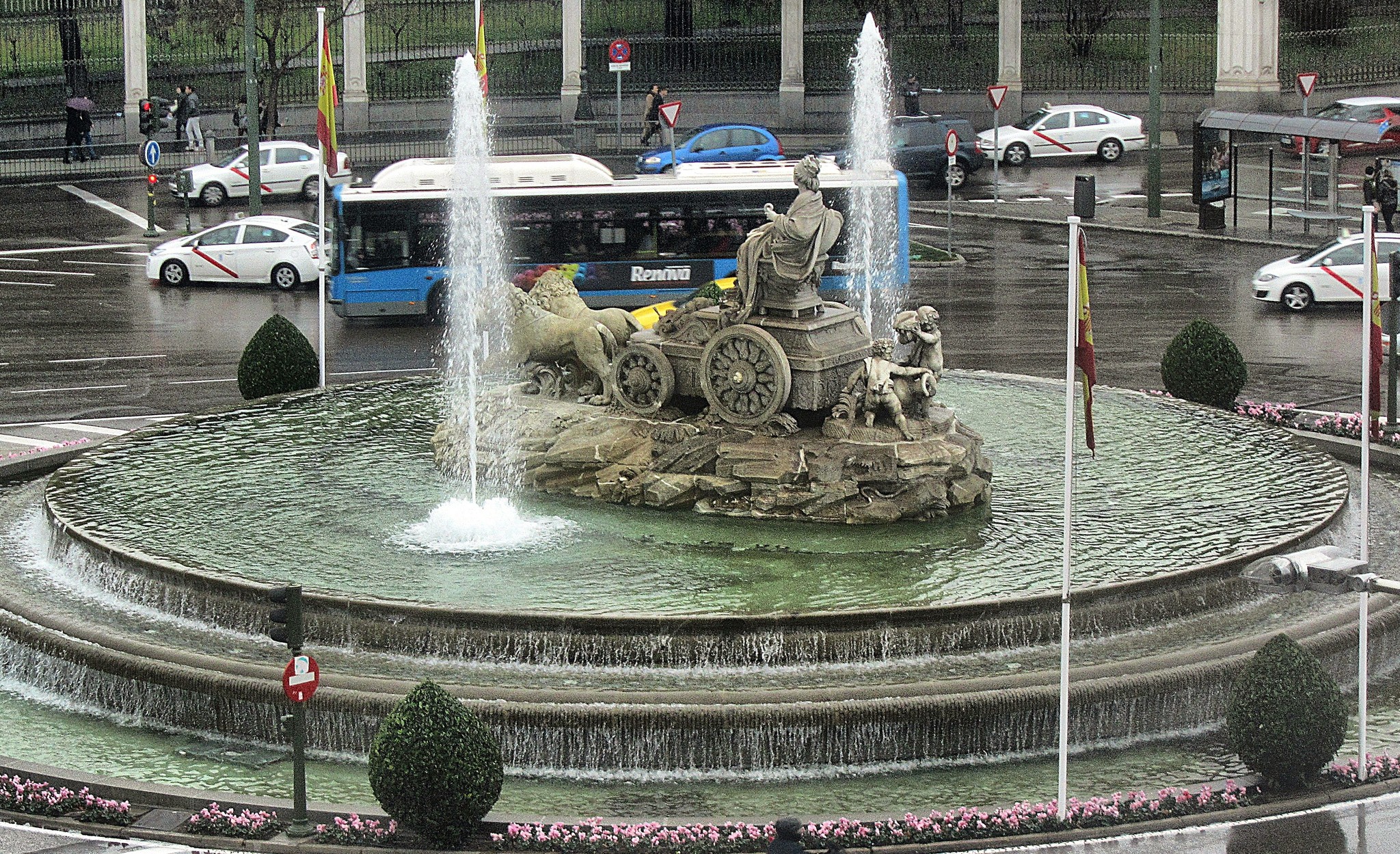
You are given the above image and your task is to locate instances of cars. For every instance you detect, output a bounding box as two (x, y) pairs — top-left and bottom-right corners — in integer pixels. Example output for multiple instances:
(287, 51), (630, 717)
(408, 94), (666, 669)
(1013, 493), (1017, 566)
(1249, 230), (1400, 313)
(142, 214), (336, 290)
(168, 139), (354, 209)
(975, 101), (1147, 167)
(1278, 103), (1400, 160)
(632, 121), (786, 175)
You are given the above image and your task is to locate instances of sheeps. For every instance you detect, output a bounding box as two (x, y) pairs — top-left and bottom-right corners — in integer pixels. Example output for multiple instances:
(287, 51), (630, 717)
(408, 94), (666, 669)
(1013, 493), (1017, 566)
(894, 373), (937, 420)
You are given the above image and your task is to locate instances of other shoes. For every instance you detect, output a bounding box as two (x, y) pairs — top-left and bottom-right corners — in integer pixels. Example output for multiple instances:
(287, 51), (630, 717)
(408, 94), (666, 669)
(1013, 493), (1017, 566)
(80, 156), (90, 162)
(63, 158), (74, 164)
(641, 139), (651, 146)
(90, 155), (103, 160)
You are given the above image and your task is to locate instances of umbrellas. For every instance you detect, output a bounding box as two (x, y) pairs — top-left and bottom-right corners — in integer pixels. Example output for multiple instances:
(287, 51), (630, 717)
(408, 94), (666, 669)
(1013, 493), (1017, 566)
(66, 97), (97, 112)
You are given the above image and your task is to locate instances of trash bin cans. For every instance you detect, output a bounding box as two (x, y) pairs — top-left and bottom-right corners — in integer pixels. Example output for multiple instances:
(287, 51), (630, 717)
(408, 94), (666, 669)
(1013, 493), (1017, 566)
(1311, 155), (1331, 197)
(1074, 173), (1095, 218)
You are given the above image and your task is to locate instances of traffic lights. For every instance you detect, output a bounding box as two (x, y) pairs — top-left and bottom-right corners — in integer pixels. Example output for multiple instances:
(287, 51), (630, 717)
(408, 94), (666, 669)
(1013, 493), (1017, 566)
(154, 96), (170, 132)
(147, 173), (157, 184)
(139, 98), (155, 135)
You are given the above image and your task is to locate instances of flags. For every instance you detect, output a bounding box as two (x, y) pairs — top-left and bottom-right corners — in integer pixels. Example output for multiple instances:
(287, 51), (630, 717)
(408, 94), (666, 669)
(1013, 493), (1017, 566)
(317, 25), (353, 174)
(1369, 226), (1385, 445)
(475, 6), (489, 102)
(1072, 233), (1099, 461)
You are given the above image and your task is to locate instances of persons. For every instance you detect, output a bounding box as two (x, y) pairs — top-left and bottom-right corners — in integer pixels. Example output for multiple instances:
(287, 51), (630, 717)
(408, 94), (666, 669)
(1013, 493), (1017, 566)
(169, 85), (208, 152)
(899, 73), (922, 116)
(896, 306), (944, 416)
(62, 94), (101, 164)
(1361, 157), (1398, 234)
(640, 84), (668, 146)
(529, 213), (767, 259)
(842, 339), (935, 440)
(735, 153), (829, 323)
(233, 93), (278, 148)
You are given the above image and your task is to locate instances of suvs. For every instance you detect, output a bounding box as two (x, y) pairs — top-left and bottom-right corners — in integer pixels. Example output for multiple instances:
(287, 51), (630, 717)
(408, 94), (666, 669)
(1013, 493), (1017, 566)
(809, 110), (985, 191)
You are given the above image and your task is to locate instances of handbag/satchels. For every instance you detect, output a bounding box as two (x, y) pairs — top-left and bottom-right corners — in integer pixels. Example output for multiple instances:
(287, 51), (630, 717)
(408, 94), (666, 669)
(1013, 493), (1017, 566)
(233, 112), (239, 127)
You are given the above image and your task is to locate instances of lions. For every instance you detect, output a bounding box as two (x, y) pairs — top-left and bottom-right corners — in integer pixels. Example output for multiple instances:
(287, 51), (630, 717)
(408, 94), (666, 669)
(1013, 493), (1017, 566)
(529, 271), (643, 395)
(481, 280), (618, 405)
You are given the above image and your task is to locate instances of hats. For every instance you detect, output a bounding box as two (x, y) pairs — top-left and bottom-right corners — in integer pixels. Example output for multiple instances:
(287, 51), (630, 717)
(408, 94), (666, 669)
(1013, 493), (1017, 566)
(908, 72), (914, 79)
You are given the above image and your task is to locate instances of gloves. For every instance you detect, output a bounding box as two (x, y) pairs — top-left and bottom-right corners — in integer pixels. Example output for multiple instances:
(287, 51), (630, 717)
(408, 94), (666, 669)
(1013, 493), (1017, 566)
(909, 91), (917, 96)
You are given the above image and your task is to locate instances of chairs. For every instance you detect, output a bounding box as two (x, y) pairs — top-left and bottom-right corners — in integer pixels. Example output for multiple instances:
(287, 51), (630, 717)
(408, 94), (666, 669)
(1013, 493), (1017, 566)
(753, 209), (845, 320)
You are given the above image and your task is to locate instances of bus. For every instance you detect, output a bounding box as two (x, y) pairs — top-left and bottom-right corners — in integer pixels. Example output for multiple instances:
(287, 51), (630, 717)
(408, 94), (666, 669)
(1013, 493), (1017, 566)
(324, 148), (913, 329)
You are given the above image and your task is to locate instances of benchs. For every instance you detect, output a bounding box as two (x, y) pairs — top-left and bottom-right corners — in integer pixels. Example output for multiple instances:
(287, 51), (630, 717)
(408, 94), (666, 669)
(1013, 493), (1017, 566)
(1287, 210), (1351, 220)
